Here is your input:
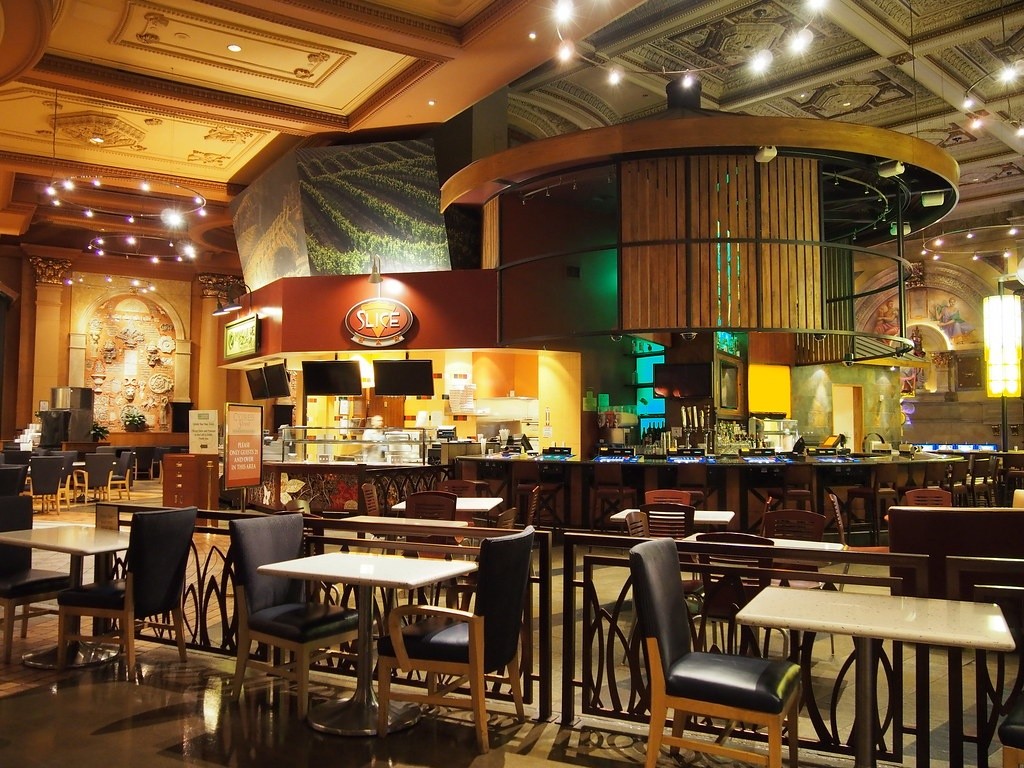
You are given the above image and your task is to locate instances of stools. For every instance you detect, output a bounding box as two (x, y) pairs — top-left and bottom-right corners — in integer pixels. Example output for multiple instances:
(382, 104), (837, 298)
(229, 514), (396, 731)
(897, 461), (944, 502)
(847, 462), (900, 546)
(588, 462), (637, 554)
(964, 452), (1024, 507)
(939, 460), (969, 507)
(459, 460), (490, 527)
(672, 461), (707, 510)
(511, 461), (559, 547)
(760, 463), (816, 536)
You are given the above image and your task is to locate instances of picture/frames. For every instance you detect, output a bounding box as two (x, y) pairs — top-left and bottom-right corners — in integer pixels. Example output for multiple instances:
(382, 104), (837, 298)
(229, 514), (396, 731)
(224, 312), (259, 360)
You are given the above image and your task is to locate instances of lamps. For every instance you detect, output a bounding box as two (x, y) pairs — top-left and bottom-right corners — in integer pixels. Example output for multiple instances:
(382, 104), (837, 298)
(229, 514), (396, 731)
(212, 287), (241, 319)
(982, 275), (1023, 453)
(223, 282), (252, 315)
(368, 253), (384, 298)
(46, 86), (209, 291)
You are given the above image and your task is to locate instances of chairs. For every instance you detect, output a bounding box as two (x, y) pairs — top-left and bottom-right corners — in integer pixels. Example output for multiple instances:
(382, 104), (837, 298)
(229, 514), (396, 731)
(746, 509), (826, 588)
(997, 689), (1024, 768)
(639, 503), (703, 580)
(56, 505), (198, 681)
(628, 537), (802, 768)
(401, 494), (454, 559)
(905, 488), (952, 507)
(437, 479), (476, 527)
(410, 491), (465, 546)
(0, 495), (70, 665)
(228, 513), (359, 721)
(620, 511), (704, 666)
(283, 500), (311, 535)
(524, 486), (540, 528)
(828, 493), (890, 592)
(271, 510), (325, 558)
(375, 524), (535, 755)
(696, 532), (773, 659)
(0, 444), (189, 517)
(361, 483), (380, 516)
(645, 489), (691, 507)
(496, 507), (518, 528)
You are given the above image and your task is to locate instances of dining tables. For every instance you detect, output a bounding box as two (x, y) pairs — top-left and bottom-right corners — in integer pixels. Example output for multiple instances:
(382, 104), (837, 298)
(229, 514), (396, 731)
(678, 532), (850, 568)
(0, 525), (132, 671)
(255, 550), (481, 737)
(339, 515), (469, 636)
(735, 585), (1016, 768)
(610, 508), (735, 524)
(27, 466), (65, 473)
(391, 497), (503, 512)
(63, 461), (117, 502)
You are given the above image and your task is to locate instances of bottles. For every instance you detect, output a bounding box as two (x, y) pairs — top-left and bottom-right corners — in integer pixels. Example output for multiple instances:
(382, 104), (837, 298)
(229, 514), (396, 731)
(642, 421), (666, 445)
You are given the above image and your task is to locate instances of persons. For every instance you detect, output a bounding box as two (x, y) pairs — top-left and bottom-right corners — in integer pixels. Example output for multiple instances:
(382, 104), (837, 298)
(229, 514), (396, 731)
(363, 415), (387, 463)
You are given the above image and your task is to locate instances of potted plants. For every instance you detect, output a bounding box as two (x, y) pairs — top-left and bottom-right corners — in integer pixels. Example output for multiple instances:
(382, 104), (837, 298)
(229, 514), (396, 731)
(89, 420), (110, 443)
(121, 409), (147, 432)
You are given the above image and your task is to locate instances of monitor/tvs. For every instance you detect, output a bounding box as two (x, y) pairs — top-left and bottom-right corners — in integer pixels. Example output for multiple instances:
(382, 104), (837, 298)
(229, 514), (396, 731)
(246, 364), (291, 400)
(301, 361), (363, 396)
(653, 362), (714, 399)
(373, 360), (434, 396)
